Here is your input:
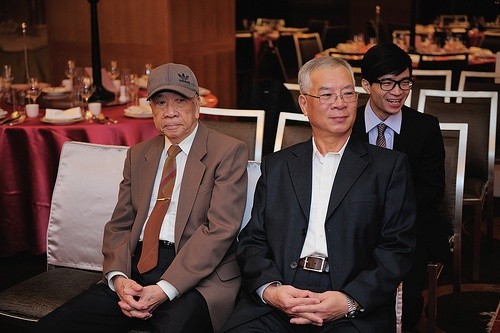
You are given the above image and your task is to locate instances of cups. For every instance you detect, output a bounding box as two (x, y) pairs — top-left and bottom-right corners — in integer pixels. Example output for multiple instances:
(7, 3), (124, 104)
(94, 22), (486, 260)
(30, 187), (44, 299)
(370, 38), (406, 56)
(25, 103), (39, 117)
(62, 79), (73, 92)
(88, 102), (102, 115)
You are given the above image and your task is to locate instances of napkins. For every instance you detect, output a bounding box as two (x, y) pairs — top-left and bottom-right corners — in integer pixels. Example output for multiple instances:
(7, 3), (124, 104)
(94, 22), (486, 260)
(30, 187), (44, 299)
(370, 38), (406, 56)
(45, 106), (81, 121)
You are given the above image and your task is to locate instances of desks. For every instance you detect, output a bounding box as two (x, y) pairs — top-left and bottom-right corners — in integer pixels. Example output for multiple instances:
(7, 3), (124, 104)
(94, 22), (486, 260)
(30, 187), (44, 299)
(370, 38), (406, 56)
(314, 49), (496, 72)
(0, 80), (217, 258)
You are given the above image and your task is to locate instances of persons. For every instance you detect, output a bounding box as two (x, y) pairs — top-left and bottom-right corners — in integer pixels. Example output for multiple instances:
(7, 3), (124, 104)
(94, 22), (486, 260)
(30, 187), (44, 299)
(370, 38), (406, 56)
(219, 55), (417, 333)
(31, 64), (248, 333)
(351, 43), (446, 333)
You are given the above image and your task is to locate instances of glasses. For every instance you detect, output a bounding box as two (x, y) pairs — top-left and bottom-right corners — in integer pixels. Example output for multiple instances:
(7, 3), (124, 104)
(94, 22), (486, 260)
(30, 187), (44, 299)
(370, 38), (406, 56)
(304, 93), (359, 104)
(373, 77), (415, 91)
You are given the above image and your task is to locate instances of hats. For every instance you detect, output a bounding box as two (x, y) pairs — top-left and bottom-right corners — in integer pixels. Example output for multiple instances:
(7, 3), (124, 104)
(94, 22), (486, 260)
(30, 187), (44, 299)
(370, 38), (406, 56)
(147, 63), (199, 101)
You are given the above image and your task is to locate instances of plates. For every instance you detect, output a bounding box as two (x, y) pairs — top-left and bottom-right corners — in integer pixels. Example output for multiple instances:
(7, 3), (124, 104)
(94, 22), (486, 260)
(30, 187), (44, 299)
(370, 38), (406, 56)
(43, 87), (67, 96)
(40, 115), (85, 124)
(123, 107), (154, 119)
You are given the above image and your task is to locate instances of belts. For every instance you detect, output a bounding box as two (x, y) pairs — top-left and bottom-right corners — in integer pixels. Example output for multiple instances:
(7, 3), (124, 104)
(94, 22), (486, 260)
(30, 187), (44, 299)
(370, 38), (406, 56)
(159, 240), (175, 247)
(299, 256), (330, 273)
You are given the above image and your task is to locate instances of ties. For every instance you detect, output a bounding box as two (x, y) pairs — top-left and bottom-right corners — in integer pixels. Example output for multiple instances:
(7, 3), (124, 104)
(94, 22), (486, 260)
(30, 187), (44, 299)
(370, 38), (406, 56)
(137, 146), (181, 274)
(376, 123), (387, 148)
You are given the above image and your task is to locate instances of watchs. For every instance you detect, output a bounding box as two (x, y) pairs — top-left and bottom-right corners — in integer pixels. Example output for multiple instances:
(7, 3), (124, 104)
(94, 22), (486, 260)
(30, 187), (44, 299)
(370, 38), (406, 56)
(346, 296), (357, 318)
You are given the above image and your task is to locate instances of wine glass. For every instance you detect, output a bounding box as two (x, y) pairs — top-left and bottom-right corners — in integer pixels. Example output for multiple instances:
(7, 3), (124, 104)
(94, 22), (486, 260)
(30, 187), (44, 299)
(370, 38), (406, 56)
(63, 58), (140, 114)
(0, 64), (41, 119)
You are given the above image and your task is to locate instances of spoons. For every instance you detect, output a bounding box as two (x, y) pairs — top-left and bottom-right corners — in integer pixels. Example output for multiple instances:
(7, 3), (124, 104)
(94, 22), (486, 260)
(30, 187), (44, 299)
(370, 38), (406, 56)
(84, 110), (96, 123)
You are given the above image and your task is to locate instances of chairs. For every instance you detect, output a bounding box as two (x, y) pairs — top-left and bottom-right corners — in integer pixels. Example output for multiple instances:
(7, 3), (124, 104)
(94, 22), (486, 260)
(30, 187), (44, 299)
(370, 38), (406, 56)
(0, 15), (500, 333)
